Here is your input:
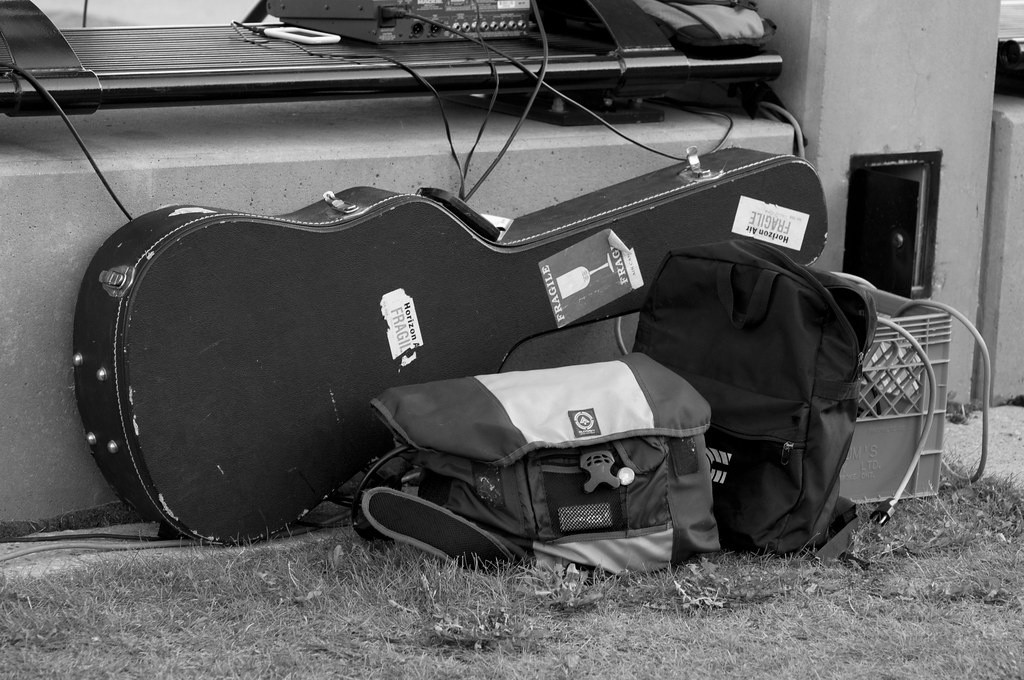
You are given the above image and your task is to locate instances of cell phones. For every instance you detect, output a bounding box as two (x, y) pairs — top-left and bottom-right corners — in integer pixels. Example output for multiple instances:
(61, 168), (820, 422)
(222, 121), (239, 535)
(264, 26), (341, 44)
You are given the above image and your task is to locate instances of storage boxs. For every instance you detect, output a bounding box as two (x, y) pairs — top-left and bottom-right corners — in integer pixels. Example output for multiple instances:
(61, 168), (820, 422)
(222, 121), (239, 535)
(839, 286), (951, 503)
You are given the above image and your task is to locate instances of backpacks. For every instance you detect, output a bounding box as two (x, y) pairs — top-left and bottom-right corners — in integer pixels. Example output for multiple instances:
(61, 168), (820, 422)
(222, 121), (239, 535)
(627, 239), (879, 563)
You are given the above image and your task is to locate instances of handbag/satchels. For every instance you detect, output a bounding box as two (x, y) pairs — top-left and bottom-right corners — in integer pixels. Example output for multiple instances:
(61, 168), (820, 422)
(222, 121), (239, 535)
(365, 351), (720, 579)
